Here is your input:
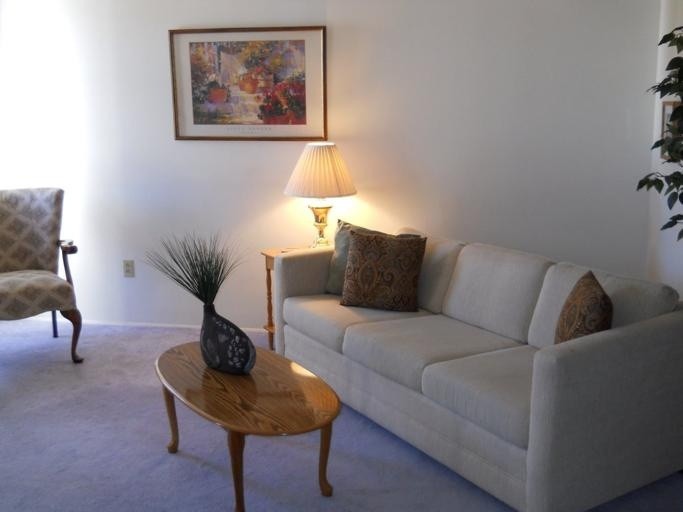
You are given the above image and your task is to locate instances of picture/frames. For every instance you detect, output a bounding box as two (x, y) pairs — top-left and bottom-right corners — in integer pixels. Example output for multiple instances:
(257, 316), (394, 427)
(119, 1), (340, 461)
(167, 23), (327, 142)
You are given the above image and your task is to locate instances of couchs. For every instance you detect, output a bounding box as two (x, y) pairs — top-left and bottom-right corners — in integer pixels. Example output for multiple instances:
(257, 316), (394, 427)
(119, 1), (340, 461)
(272, 227), (683, 511)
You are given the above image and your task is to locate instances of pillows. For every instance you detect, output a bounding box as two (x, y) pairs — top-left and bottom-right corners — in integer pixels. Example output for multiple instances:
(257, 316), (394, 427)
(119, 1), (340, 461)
(556, 271), (613, 347)
(325, 217), (428, 314)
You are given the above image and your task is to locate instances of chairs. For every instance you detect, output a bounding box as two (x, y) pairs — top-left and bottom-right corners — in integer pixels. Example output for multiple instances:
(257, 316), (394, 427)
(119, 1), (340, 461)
(0, 185), (83, 365)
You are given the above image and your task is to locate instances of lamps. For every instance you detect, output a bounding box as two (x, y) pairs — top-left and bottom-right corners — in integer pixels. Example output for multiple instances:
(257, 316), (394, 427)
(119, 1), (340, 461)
(284, 142), (359, 253)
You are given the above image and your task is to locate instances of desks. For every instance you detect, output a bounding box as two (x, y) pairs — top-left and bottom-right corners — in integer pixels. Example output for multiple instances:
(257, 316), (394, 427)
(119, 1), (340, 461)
(260, 246), (312, 350)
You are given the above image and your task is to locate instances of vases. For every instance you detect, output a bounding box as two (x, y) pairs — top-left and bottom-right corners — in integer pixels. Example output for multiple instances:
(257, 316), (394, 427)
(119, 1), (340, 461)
(199, 303), (255, 375)
